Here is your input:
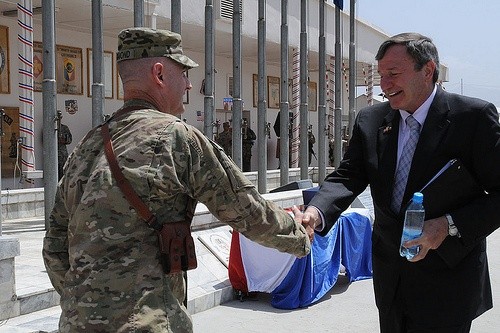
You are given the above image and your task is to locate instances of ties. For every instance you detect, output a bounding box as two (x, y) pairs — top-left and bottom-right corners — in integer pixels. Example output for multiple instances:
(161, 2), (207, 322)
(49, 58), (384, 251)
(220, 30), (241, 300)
(393, 116), (419, 215)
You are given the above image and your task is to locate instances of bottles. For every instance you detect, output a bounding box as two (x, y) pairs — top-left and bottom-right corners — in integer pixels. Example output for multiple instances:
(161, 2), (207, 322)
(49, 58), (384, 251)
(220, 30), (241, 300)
(398, 192), (426, 261)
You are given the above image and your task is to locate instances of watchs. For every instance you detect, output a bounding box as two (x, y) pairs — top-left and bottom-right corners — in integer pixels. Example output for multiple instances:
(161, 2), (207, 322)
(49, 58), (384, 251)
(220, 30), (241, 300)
(446, 213), (459, 236)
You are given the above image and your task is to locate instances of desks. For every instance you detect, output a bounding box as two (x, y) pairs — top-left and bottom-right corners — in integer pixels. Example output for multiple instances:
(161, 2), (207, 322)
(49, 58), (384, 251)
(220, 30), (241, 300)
(229, 207), (374, 309)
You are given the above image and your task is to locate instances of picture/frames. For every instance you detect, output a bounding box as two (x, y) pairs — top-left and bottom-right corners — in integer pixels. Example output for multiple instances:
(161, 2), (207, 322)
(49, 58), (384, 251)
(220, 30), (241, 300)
(0, 25), (11, 94)
(87, 47), (114, 98)
(308, 81), (317, 111)
(34, 41), (43, 91)
(183, 69), (189, 104)
(288, 79), (293, 109)
(266, 76), (280, 109)
(57, 45), (83, 95)
(252, 74), (258, 107)
(116, 72), (124, 100)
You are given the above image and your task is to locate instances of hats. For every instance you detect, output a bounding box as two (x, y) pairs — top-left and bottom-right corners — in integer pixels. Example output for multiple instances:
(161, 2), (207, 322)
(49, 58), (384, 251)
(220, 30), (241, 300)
(223, 122), (230, 126)
(116, 27), (199, 71)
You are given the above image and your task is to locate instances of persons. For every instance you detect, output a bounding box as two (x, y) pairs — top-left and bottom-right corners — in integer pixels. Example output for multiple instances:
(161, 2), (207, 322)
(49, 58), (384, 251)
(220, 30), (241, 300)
(328, 140), (334, 167)
(55, 110), (73, 179)
(308, 126), (317, 168)
(42, 27), (315, 333)
(241, 116), (256, 172)
(302, 33), (500, 333)
(219, 121), (233, 158)
(273, 109), (292, 169)
